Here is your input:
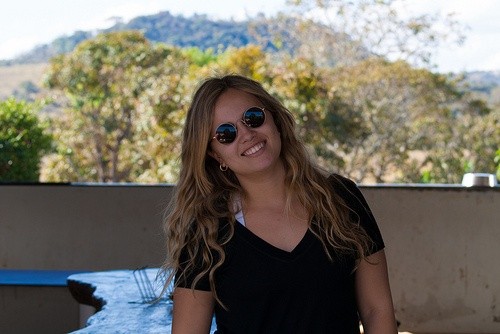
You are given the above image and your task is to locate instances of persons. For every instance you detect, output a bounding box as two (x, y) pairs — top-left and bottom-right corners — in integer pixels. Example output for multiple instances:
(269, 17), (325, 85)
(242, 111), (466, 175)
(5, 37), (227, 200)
(162, 72), (398, 334)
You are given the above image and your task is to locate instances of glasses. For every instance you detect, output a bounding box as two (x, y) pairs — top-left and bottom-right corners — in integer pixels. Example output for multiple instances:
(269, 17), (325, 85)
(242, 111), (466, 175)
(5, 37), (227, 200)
(213, 106), (267, 145)
